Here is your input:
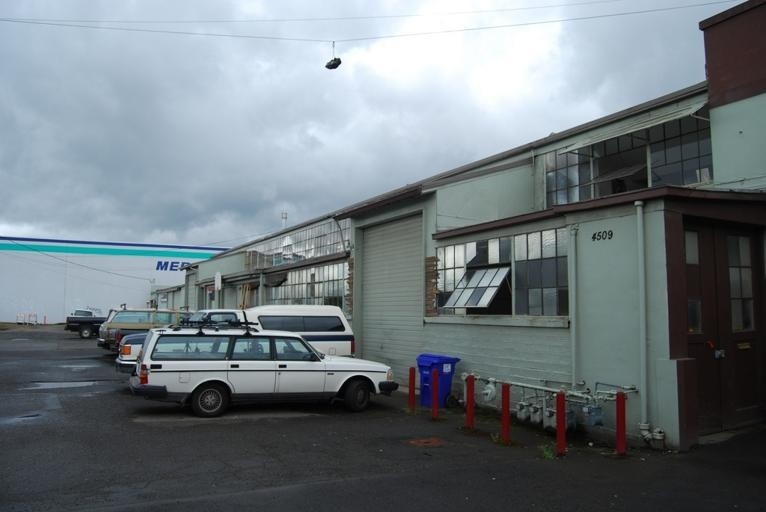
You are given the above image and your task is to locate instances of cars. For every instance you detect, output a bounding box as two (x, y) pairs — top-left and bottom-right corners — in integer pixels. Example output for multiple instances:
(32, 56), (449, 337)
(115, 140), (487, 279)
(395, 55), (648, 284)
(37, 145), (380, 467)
(114, 327), (149, 352)
(96, 309), (118, 349)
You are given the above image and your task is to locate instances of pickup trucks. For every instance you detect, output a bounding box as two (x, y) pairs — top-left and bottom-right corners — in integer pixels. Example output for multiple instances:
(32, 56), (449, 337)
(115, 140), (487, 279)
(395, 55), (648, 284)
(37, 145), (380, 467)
(65, 308), (117, 339)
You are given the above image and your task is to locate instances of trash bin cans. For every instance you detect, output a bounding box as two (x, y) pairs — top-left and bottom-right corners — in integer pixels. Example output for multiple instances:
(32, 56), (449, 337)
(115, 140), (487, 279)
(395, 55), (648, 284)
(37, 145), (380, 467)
(416, 354), (461, 409)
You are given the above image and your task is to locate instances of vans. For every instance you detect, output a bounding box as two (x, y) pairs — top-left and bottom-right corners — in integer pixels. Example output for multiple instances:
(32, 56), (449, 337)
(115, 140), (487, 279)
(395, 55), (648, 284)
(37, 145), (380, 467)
(104, 309), (194, 351)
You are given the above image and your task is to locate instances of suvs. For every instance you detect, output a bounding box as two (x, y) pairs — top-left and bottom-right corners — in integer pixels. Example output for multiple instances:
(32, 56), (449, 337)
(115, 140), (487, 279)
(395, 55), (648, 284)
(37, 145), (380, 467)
(126, 319), (399, 417)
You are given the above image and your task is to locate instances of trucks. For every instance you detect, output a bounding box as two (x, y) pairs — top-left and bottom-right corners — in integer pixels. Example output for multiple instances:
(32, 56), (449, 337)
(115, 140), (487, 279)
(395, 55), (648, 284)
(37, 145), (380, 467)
(115, 304), (356, 372)
